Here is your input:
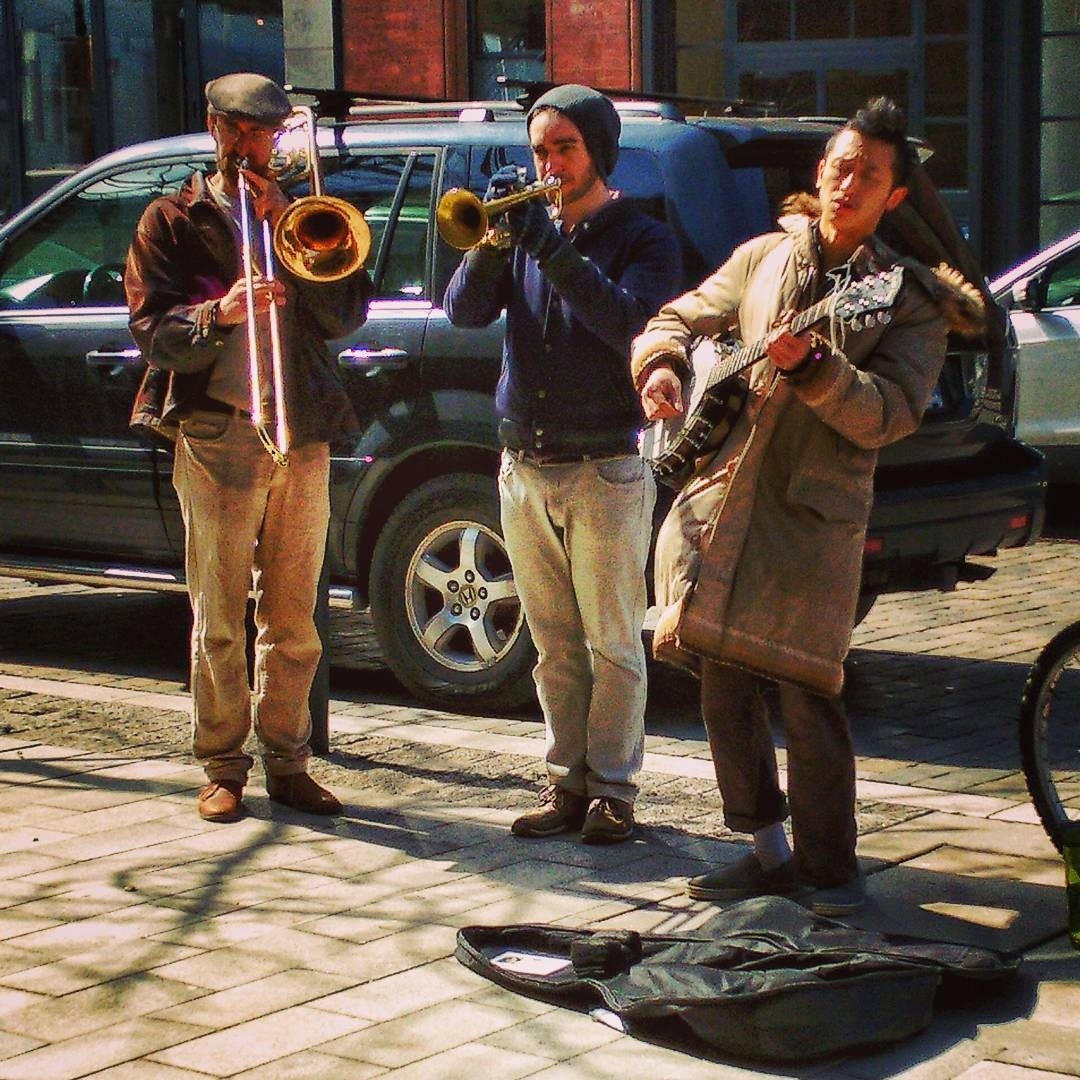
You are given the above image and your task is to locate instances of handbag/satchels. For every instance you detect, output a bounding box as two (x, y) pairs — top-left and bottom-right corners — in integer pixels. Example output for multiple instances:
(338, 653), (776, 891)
(453, 893), (1022, 1066)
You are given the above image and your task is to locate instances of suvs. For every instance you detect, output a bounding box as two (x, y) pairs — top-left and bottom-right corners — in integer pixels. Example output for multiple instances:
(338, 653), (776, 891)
(0, 72), (1054, 721)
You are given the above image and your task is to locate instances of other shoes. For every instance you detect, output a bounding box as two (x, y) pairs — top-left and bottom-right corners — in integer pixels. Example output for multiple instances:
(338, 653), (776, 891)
(814, 883), (866, 914)
(688, 851), (803, 899)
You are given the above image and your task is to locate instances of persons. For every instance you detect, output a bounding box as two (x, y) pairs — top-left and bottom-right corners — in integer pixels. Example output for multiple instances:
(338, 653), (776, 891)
(631, 97), (991, 920)
(442, 84), (684, 844)
(122, 68), (373, 823)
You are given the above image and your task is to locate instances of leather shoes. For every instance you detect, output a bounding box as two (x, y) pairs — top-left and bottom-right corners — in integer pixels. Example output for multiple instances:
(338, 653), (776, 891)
(198, 779), (240, 822)
(266, 770), (342, 816)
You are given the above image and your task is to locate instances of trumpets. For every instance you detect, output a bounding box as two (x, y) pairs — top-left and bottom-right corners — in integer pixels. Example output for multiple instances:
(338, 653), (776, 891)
(436, 173), (563, 252)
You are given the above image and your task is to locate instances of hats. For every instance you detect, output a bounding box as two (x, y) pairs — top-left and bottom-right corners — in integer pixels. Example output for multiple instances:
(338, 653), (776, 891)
(527, 83), (621, 179)
(206, 73), (292, 121)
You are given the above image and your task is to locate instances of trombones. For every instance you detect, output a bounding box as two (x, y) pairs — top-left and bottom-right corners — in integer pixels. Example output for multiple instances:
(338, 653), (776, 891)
(235, 105), (373, 470)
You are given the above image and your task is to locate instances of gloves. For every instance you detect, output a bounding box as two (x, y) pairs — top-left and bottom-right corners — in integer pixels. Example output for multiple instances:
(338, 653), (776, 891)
(504, 197), (560, 263)
(483, 164), (518, 227)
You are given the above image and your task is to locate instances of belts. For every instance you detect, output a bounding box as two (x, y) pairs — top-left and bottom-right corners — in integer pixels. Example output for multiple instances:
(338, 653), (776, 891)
(197, 398), (248, 419)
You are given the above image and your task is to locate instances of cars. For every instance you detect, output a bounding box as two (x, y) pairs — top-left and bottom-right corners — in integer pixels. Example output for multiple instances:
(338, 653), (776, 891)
(989, 228), (1080, 488)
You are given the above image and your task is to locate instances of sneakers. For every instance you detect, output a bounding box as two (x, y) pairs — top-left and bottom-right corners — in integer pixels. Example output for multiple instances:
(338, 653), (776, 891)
(582, 798), (632, 842)
(511, 788), (584, 837)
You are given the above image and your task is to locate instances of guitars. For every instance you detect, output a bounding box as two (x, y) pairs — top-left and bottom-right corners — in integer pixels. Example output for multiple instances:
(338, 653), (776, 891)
(634, 262), (907, 478)
